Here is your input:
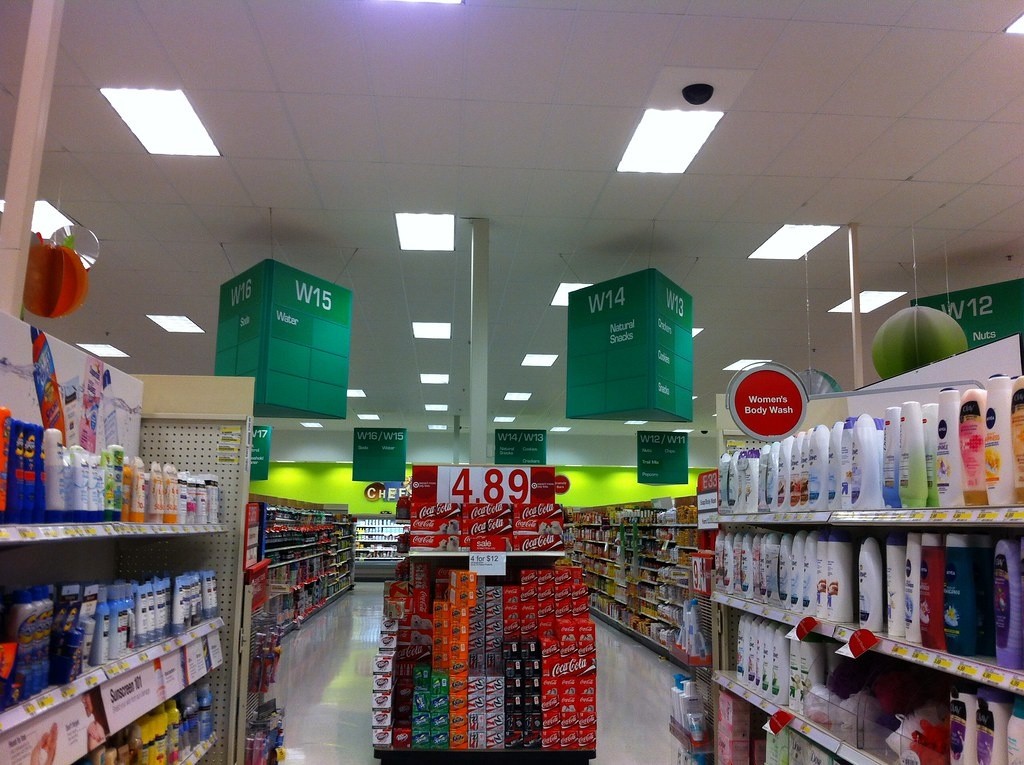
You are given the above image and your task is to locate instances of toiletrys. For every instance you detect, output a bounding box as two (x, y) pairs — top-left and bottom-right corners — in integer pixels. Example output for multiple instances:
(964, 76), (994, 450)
(717, 372), (1024, 765)
(0, 406), (220, 765)
(672, 598), (717, 765)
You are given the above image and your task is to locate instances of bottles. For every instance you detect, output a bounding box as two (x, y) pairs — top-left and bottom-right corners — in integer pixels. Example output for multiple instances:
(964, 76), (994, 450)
(73, 680), (215, 765)
(671, 600), (710, 765)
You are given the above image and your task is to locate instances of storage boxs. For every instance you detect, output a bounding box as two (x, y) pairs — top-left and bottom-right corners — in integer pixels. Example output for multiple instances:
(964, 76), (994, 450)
(373, 502), (598, 749)
(717, 688), (843, 765)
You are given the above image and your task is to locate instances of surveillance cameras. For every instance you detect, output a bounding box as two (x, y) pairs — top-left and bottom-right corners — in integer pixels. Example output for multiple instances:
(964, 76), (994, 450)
(702, 430), (709, 435)
(682, 84), (714, 106)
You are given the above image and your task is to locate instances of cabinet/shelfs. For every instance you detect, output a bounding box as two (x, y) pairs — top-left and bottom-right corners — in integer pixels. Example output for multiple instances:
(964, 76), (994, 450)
(572, 507), (698, 675)
(0, 413), (253, 765)
(354, 514), (410, 580)
(668, 393), (1024, 765)
(264, 505), (357, 638)
